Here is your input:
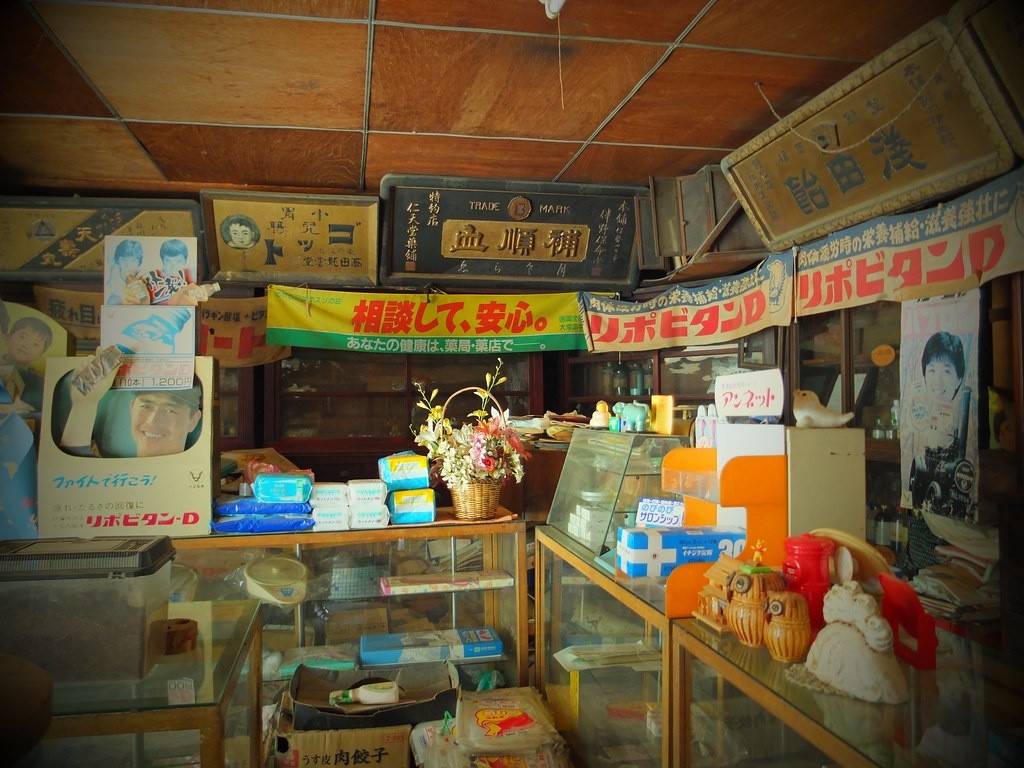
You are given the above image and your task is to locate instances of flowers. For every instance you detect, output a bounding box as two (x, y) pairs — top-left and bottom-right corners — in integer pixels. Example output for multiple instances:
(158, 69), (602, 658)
(408, 358), (532, 495)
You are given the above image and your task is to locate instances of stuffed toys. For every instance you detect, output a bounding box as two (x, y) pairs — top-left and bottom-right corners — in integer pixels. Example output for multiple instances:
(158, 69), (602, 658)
(793, 388), (854, 427)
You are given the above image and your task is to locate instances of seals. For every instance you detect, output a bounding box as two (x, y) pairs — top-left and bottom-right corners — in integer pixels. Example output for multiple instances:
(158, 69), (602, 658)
(792, 388), (855, 429)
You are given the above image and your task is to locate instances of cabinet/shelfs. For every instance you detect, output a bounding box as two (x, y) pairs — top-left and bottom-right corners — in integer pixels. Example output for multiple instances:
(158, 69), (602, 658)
(546, 427), (690, 555)
(169, 517), (528, 754)
(788, 299), (902, 464)
(562, 327), (774, 413)
(535, 526), (670, 768)
(672, 617), (1023, 768)
(634, 164), (769, 288)
(264, 346), (545, 452)
(214, 366), (255, 460)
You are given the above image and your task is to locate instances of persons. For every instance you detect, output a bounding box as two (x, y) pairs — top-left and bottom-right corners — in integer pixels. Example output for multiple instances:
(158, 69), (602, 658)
(908, 332), (977, 508)
(0, 300), (51, 456)
(106, 239), (193, 306)
(59, 347), (201, 458)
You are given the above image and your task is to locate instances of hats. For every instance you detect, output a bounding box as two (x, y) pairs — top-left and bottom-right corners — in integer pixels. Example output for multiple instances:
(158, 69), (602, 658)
(131, 384), (201, 407)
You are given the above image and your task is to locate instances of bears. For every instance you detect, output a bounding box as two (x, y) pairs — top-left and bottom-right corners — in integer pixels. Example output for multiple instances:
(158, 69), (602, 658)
(612, 399), (650, 433)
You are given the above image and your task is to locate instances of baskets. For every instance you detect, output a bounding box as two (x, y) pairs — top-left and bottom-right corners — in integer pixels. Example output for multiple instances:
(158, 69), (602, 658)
(440, 386), (507, 520)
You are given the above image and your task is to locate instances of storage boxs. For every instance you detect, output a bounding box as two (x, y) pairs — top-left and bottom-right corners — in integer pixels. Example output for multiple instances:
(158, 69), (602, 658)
(0, 535), (514, 768)
(616, 526), (746, 577)
